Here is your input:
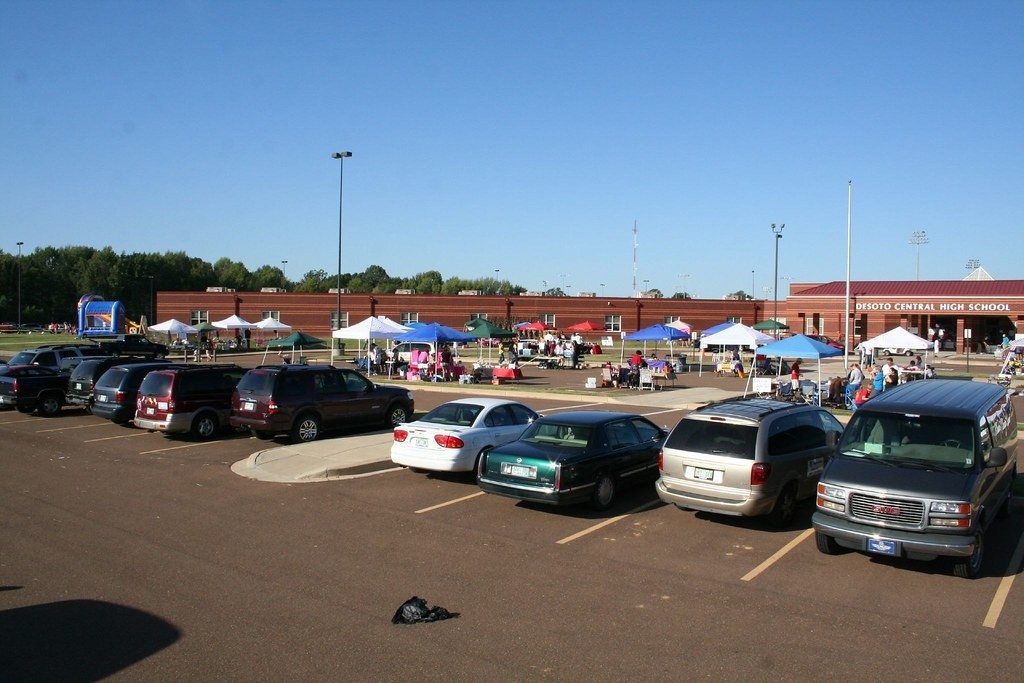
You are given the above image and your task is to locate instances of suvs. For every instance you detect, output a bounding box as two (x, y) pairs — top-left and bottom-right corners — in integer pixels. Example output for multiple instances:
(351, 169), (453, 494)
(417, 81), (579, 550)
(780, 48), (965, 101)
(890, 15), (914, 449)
(134, 362), (248, 442)
(90, 362), (199, 427)
(0, 343), (171, 419)
(228, 364), (414, 445)
(655, 397), (845, 529)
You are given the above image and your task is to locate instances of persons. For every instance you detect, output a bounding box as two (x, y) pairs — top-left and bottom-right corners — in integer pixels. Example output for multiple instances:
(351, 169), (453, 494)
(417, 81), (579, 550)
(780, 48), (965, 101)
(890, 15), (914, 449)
(172, 330), (250, 362)
(628, 338), (936, 411)
(932, 332), (1010, 358)
(499, 331), (584, 370)
(64, 322), (77, 335)
(369, 341), (457, 383)
(48, 322), (59, 335)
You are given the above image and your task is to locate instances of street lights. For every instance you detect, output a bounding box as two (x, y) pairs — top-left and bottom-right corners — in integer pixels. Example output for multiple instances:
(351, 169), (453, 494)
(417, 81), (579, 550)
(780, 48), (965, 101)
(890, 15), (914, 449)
(495, 270), (499, 281)
(600, 284), (605, 296)
(281, 260), (288, 275)
(908, 229), (930, 281)
(16, 241), (24, 327)
(332, 151), (353, 349)
(771, 223), (786, 338)
(643, 279), (649, 292)
(752, 270), (755, 300)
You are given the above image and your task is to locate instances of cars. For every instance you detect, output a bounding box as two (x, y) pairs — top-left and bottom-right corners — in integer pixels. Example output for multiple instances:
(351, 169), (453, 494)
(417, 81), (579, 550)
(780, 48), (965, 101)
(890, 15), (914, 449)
(0, 321), (66, 334)
(390, 397), (568, 485)
(476, 410), (670, 513)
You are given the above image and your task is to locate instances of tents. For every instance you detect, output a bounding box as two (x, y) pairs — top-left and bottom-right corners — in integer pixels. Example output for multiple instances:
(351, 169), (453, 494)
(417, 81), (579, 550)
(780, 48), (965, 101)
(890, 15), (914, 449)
(382, 317), (415, 348)
(211, 314), (259, 349)
(664, 320), (693, 335)
(388, 322), (478, 382)
(329, 315), (408, 377)
(148, 319), (200, 362)
(699, 323), (776, 380)
(567, 320), (608, 336)
(618, 323), (695, 366)
(255, 316), (292, 348)
(754, 319), (792, 340)
(468, 323), (517, 361)
(702, 320), (736, 336)
(260, 331), (330, 364)
(859, 325), (936, 380)
(743, 334), (845, 408)
(518, 320), (555, 340)
(192, 321), (220, 340)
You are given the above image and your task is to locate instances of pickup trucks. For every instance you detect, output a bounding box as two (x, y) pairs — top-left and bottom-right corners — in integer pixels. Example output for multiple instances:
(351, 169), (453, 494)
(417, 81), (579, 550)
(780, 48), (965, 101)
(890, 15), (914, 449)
(92, 334), (170, 361)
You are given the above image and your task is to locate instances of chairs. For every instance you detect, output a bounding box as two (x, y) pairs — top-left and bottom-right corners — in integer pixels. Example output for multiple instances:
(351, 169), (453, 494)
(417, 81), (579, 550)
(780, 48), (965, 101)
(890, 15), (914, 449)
(779, 381), (822, 407)
(712, 354), (719, 362)
(639, 368), (655, 392)
(602, 368), (620, 389)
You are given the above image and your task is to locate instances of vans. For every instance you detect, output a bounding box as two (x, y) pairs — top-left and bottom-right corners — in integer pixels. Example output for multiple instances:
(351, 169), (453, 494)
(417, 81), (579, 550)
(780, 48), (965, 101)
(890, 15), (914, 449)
(812, 379), (1019, 579)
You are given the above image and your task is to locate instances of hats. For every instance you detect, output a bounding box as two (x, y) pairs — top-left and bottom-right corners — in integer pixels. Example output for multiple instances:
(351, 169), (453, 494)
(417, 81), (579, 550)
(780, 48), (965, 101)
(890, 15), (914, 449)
(797, 358), (802, 361)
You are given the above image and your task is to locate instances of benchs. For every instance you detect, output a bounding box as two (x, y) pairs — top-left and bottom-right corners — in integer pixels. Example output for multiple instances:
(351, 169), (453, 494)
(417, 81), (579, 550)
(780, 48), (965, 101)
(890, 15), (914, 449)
(431, 417), (471, 424)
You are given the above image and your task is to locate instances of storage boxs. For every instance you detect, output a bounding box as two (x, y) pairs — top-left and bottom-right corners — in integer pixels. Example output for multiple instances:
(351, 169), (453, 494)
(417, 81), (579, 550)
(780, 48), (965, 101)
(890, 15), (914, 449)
(585, 377), (596, 388)
(1015, 368), (1022, 375)
(491, 378), (502, 385)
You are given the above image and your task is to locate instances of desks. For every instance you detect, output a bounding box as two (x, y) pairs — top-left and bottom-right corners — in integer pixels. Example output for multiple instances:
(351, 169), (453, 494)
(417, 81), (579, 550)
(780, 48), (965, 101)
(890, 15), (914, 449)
(626, 358), (669, 371)
(601, 371), (635, 390)
(713, 364), (747, 378)
(535, 356), (564, 372)
(409, 363), (465, 381)
(652, 372), (678, 390)
(902, 369), (924, 381)
(988, 378), (1011, 388)
(474, 369), (524, 386)
(757, 365), (774, 375)
